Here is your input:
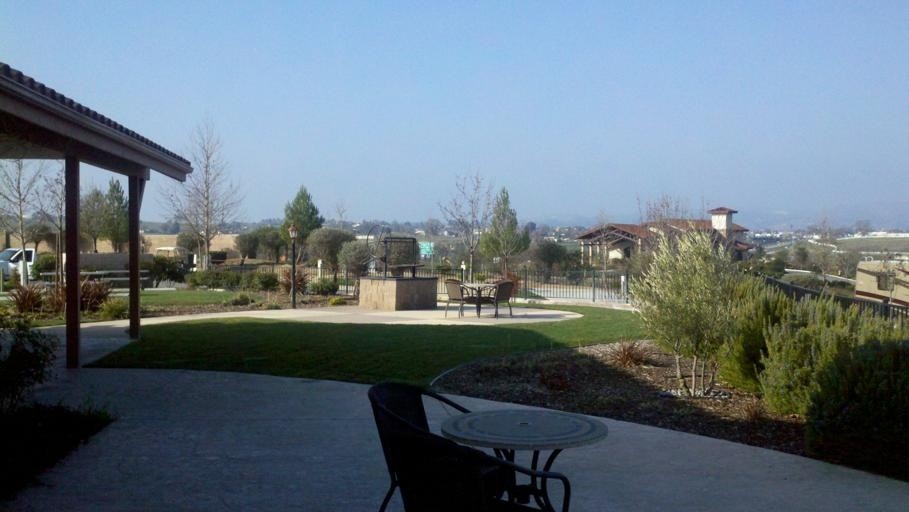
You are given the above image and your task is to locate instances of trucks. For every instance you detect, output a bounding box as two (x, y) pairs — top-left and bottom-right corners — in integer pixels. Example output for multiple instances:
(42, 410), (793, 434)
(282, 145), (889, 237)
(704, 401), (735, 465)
(0, 248), (39, 282)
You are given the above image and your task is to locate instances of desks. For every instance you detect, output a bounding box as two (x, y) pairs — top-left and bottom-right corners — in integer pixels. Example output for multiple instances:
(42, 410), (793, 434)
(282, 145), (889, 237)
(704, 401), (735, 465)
(441, 409), (608, 512)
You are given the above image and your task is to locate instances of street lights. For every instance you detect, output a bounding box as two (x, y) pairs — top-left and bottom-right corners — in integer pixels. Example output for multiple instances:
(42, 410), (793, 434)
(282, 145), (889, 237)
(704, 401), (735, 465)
(287, 222), (300, 308)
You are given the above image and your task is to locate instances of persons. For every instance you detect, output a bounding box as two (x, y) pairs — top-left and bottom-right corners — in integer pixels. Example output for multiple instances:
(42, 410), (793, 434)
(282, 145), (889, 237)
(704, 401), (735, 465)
(460, 259), (469, 283)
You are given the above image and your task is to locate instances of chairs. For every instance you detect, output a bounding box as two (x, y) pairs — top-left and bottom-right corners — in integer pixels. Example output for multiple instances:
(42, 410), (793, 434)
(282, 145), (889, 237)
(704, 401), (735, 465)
(444, 279), (514, 319)
(367, 382), (571, 511)
